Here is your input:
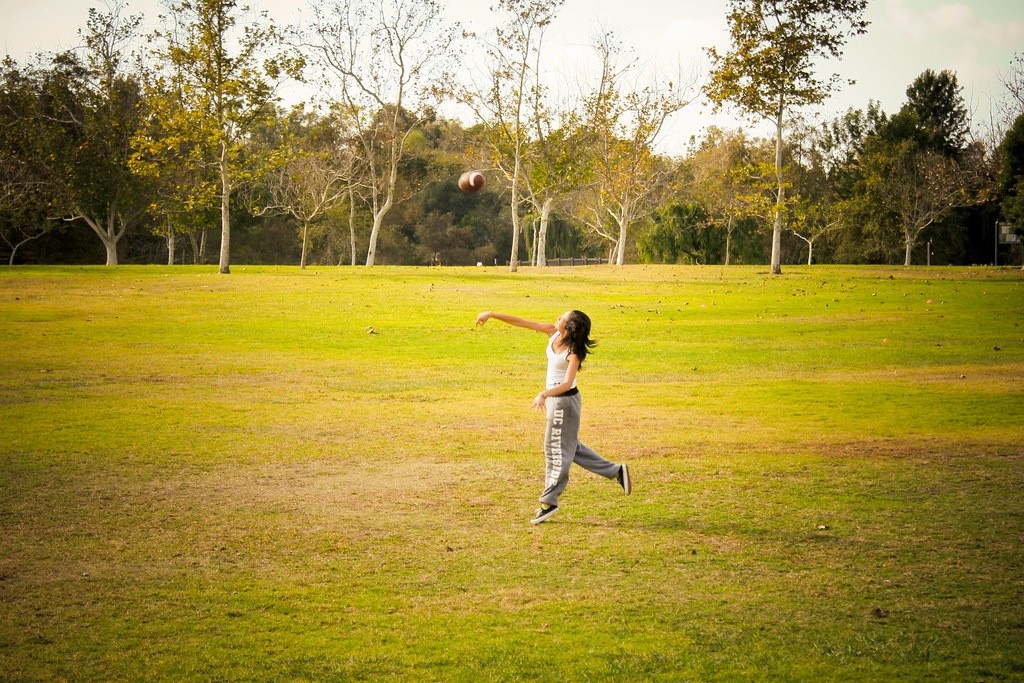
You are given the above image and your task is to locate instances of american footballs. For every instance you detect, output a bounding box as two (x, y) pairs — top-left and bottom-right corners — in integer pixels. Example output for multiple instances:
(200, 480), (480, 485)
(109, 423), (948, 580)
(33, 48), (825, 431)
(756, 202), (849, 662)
(457, 172), (485, 192)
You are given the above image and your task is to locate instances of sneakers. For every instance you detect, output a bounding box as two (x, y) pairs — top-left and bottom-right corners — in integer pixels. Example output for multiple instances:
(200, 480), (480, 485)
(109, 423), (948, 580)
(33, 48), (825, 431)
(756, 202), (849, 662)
(617, 464), (631, 496)
(531, 505), (559, 524)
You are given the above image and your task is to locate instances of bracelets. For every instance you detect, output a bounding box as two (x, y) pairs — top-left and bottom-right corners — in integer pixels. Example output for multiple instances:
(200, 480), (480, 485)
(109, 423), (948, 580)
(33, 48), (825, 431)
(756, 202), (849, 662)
(539, 392), (547, 399)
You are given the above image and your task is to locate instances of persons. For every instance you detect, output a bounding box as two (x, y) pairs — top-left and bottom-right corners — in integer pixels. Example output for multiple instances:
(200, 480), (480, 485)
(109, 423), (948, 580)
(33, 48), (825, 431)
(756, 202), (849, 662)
(474, 308), (633, 524)
